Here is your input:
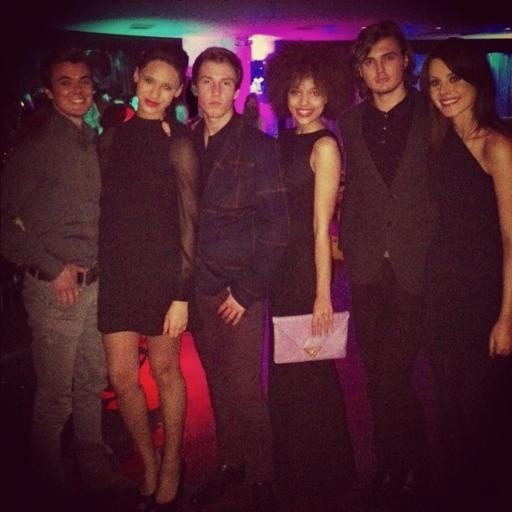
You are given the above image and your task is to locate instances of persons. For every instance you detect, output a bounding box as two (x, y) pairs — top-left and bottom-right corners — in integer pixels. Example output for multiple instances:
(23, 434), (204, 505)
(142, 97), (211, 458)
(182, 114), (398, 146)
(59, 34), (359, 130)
(1, 39), (268, 127)
(256, 42), (363, 510)
(178, 47), (296, 509)
(1, 36), (126, 483)
(323, 18), (447, 510)
(97, 45), (200, 511)
(413, 38), (510, 510)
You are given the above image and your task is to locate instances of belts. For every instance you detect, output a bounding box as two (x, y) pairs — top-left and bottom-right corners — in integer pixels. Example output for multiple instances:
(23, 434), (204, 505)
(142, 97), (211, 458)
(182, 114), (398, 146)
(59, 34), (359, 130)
(27, 266), (97, 286)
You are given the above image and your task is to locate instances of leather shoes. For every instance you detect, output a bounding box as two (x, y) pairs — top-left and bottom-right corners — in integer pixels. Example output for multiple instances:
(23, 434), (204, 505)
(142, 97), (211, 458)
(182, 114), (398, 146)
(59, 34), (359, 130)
(248, 472), (278, 512)
(136, 449), (187, 512)
(190, 456), (238, 501)
(369, 461), (427, 512)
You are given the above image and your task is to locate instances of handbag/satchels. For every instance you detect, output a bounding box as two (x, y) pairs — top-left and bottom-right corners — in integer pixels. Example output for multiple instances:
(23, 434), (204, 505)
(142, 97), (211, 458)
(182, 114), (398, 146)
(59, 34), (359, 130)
(272, 310), (350, 364)
